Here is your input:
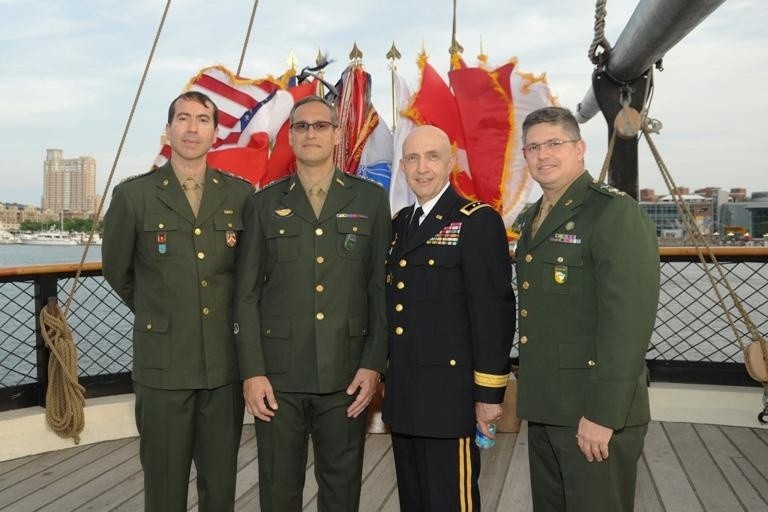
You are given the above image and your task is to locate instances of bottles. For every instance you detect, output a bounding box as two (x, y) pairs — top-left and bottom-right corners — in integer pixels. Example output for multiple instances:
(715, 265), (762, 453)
(476, 422), (496, 448)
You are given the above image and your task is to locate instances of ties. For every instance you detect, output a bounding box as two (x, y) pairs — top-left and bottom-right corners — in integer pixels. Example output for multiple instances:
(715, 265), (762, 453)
(410, 207), (424, 235)
(184, 180), (201, 217)
(531, 200), (551, 241)
(309, 186), (322, 218)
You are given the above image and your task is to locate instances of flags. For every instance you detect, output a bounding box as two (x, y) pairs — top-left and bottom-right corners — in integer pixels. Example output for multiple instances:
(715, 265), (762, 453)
(150, 56), (560, 225)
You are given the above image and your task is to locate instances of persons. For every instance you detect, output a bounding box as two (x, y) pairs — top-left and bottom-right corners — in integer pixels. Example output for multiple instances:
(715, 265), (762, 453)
(100, 90), (260, 512)
(234, 92), (393, 511)
(512, 105), (663, 512)
(381, 124), (517, 512)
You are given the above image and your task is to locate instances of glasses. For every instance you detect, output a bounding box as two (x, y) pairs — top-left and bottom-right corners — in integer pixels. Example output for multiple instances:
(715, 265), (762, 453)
(291, 121), (337, 131)
(521, 139), (576, 153)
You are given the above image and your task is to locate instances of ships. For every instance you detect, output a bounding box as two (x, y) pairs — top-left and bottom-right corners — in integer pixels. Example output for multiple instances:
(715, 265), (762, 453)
(0, 232), (102, 246)
